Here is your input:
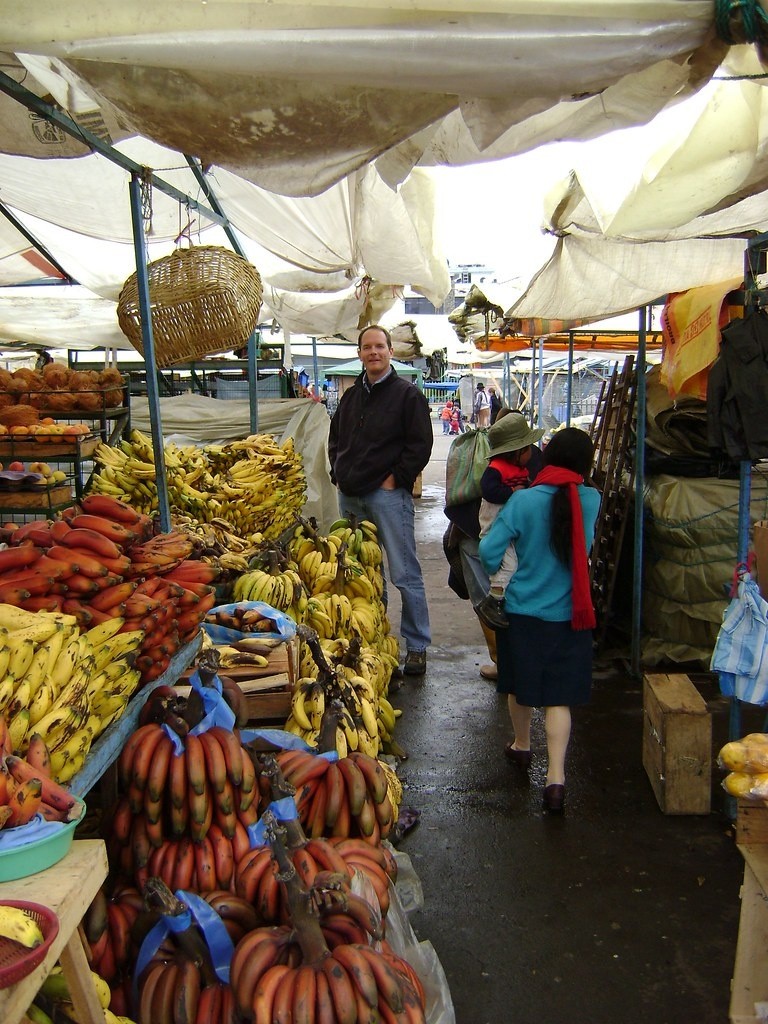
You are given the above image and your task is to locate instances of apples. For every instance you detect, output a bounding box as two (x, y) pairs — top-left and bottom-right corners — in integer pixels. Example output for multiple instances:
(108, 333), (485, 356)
(0, 417), (90, 484)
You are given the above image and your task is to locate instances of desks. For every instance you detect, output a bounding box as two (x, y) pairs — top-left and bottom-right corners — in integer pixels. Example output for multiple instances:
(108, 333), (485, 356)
(0, 839), (110, 1024)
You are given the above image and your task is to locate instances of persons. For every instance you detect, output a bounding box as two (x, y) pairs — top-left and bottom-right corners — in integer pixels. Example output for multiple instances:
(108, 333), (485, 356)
(327, 326), (434, 678)
(442, 383), (601, 807)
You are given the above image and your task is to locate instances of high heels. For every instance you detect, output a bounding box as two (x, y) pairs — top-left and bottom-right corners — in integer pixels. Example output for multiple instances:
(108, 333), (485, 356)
(543, 784), (565, 810)
(504, 740), (532, 772)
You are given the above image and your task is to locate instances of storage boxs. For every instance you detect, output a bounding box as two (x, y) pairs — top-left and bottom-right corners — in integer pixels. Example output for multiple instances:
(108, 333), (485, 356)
(735, 798), (767, 844)
(643, 672), (712, 816)
(173, 638), (301, 730)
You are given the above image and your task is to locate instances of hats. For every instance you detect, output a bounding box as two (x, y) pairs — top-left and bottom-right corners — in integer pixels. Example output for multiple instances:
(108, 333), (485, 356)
(446, 402), (453, 407)
(477, 383), (485, 389)
(483, 413), (545, 459)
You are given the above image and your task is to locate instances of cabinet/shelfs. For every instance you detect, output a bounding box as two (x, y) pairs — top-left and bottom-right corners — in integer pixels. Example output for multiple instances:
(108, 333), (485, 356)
(0, 374), (133, 525)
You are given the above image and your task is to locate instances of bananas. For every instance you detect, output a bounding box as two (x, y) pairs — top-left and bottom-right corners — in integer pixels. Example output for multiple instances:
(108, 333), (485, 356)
(0, 430), (430, 1024)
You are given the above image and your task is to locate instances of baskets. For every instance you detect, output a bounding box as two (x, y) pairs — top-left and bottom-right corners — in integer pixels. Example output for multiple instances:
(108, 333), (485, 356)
(0, 900), (59, 990)
(117, 247), (263, 371)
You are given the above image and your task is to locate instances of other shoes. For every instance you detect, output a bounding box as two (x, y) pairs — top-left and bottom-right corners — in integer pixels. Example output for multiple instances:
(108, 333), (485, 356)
(480, 664), (498, 678)
(405, 651), (426, 674)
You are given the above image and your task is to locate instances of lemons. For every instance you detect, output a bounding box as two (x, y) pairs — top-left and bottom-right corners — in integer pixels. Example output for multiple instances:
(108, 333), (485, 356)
(719, 733), (768, 806)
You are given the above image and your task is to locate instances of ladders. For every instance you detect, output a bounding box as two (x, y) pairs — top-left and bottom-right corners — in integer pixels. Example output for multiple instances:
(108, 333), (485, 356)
(588, 355), (648, 626)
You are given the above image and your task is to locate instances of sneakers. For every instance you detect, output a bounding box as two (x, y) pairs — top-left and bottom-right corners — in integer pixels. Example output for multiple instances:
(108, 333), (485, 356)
(472, 594), (510, 632)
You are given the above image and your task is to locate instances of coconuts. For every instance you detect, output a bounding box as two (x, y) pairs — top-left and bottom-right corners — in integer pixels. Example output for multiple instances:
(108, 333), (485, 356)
(0, 364), (124, 410)
